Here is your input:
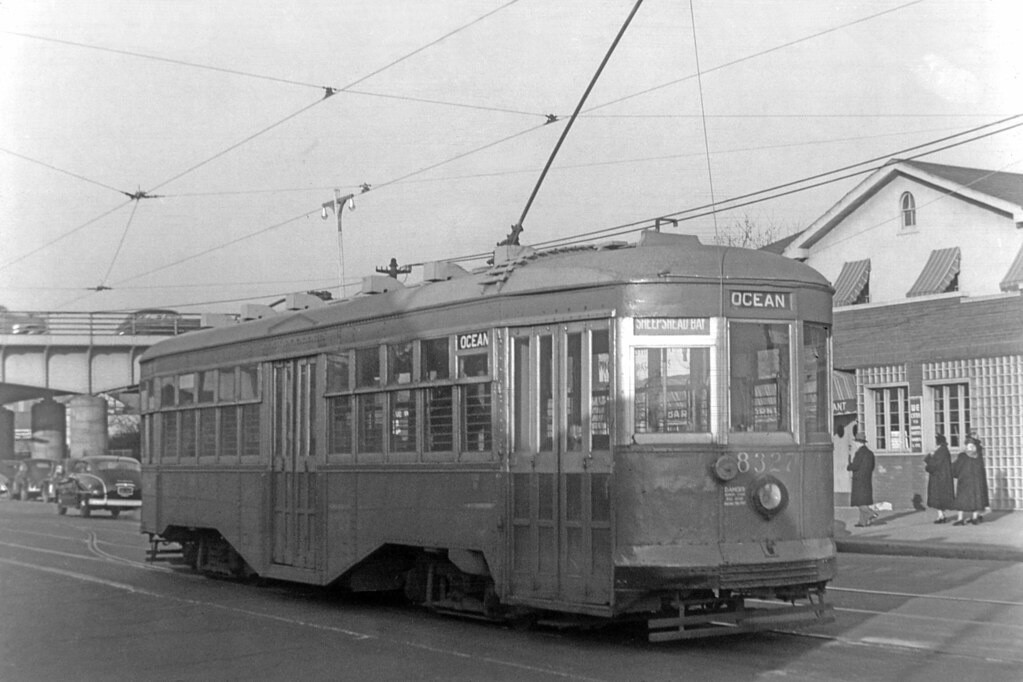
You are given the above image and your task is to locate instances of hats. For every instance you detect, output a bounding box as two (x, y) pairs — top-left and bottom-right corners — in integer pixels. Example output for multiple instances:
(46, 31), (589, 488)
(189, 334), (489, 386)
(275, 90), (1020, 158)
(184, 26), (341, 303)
(965, 436), (980, 447)
(852, 432), (868, 443)
(935, 433), (948, 446)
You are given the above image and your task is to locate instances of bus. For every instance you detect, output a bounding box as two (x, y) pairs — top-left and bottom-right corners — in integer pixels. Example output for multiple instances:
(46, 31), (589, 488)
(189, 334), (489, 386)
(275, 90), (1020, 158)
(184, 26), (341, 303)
(138, 231), (837, 644)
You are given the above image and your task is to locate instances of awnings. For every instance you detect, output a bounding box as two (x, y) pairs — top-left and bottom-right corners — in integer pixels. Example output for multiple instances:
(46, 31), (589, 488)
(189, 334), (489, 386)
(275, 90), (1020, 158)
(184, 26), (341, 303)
(1000, 245), (1023, 290)
(832, 260), (870, 306)
(906, 248), (959, 297)
(833, 370), (856, 417)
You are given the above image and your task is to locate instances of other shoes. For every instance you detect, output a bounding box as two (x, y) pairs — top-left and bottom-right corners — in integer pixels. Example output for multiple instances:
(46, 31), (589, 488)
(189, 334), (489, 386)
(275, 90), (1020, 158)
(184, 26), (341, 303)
(967, 519), (977, 524)
(854, 523), (864, 527)
(977, 516), (983, 522)
(868, 514), (878, 521)
(953, 520), (963, 527)
(934, 518), (946, 524)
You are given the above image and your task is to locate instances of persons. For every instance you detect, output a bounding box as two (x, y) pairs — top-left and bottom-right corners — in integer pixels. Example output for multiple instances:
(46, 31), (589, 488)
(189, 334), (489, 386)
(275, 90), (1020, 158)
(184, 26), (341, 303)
(951, 433), (989, 525)
(847, 432), (878, 527)
(924, 433), (954, 523)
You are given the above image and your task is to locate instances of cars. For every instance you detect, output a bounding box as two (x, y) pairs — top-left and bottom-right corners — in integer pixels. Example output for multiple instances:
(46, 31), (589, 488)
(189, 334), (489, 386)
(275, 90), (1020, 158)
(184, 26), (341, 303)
(12, 458), (57, 501)
(0, 304), (50, 336)
(0, 459), (22, 499)
(116, 308), (185, 335)
(43, 459), (77, 502)
(55, 455), (142, 518)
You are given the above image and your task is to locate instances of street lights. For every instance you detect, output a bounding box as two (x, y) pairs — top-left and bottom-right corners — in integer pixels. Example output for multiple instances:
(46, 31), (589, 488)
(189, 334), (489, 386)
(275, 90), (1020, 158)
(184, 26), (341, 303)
(321, 187), (355, 299)
